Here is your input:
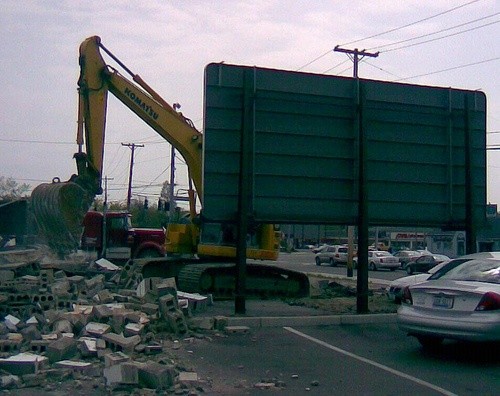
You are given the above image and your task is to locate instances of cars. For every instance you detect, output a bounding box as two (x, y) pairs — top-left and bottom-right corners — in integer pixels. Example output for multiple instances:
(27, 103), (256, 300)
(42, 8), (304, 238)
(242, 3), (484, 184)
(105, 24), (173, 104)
(405, 252), (451, 274)
(416, 249), (432, 257)
(387, 257), (457, 310)
(394, 249), (500, 347)
(351, 250), (401, 272)
(390, 251), (421, 270)
(315, 245), (350, 267)
(312, 245), (326, 254)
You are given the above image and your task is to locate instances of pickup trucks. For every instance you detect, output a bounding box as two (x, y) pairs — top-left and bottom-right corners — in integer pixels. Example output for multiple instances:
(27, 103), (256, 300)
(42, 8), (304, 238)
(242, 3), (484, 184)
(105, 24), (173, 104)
(344, 243), (371, 257)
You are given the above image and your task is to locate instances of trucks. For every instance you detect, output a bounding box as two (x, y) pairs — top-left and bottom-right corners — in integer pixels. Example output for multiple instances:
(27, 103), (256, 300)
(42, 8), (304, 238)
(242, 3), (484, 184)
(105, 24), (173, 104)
(73, 208), (168, 267)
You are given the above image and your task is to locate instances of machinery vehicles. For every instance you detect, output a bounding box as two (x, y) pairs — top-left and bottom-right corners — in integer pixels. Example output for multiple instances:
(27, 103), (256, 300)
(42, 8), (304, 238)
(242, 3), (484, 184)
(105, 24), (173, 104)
(28, 30), (316, 301)
